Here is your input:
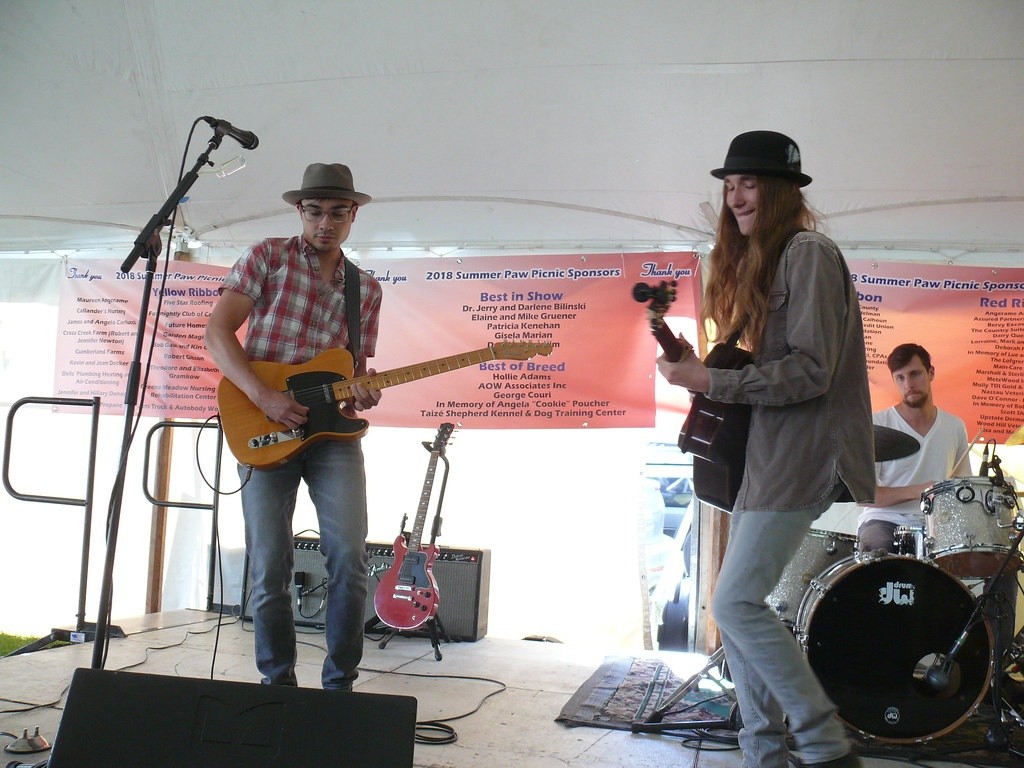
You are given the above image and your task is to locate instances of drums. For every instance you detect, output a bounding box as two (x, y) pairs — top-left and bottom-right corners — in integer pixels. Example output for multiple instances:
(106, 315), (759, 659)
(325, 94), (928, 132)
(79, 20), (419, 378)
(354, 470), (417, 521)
(918, 476), (1023, 582)
(793, 549), (998, 746)
(763, 528), (862, 635)
(893, 524), (929, 559)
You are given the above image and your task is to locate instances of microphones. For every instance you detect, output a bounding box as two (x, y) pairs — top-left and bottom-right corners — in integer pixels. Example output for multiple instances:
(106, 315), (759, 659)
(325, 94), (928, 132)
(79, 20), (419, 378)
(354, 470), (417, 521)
(926, 665), (949, 688)
(980, 444), (988, 476)
(294, 572), (305, 612)
(203, 116), (259, 150)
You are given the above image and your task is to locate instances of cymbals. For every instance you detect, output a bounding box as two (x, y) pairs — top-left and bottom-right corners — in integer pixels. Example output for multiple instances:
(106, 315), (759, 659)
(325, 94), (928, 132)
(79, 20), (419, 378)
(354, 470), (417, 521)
(866, 421), (922, 464)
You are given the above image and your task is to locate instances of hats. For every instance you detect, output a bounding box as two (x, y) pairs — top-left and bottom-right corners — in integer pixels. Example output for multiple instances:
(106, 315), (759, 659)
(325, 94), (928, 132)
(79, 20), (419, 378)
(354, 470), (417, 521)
(282, 162), (373, 207)
(710, 130), (813, 188)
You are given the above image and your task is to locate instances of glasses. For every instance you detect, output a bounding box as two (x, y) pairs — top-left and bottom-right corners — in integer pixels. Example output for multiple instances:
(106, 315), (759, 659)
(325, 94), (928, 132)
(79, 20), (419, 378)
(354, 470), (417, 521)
(299, 200), (354, 223)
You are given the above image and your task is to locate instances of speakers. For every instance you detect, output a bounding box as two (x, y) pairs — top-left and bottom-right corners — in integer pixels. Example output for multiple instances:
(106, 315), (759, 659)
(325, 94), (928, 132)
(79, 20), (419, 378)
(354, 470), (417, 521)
(47, 668), (418, 768)
(362, 543), (491, 643)
(240, 537), (329, 629)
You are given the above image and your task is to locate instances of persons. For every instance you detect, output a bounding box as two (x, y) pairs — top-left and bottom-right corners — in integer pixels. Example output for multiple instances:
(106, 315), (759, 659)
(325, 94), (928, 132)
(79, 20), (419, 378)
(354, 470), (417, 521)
(204, 162), (384, 692)
(653, 130), (877, 768)
(856, 343), (1018, 661)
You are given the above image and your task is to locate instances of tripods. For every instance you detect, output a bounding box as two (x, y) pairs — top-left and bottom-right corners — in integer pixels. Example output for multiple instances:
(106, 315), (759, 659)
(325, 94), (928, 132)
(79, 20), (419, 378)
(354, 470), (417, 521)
(379, 441), (450, 661)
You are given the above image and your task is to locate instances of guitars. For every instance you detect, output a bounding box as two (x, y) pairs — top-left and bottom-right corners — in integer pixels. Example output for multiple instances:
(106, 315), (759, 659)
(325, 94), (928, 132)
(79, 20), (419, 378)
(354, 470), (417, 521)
(373, 421), (461, 631)
(632, 272), (754, 514)
(215, 338), (554, 472)
